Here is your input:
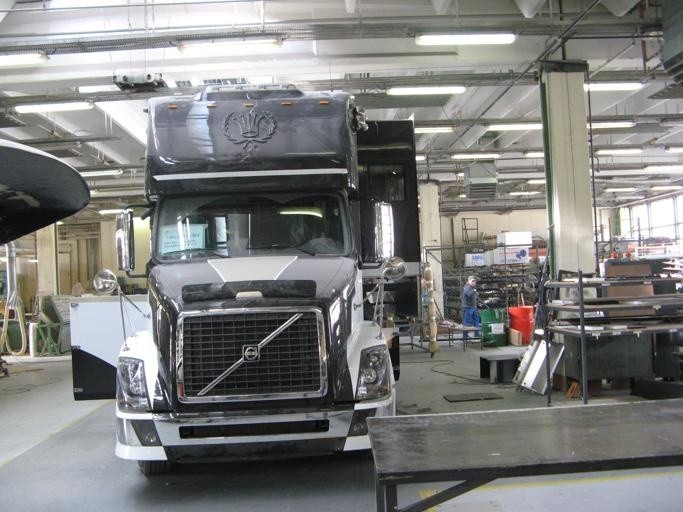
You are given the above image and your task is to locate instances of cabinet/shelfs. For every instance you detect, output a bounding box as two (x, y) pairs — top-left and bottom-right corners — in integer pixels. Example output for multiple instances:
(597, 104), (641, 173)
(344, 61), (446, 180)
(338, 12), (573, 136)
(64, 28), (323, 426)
(415, 241), (552, 352)
(541, 271), (682, 408)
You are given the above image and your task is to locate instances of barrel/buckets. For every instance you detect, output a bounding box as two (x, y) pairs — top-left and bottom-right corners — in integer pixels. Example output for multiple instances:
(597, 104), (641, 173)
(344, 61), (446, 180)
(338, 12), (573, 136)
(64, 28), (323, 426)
(508, 305), (535, 347)
(479, 308), (509, 348)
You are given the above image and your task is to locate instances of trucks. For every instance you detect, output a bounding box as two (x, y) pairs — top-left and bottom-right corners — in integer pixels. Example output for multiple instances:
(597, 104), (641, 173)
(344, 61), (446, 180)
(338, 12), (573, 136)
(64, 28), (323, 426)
(67, 81), (430, 478)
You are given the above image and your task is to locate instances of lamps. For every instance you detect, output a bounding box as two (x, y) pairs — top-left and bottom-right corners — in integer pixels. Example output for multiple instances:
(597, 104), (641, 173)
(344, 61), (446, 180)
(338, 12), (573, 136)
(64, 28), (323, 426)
(660, 118), (682, 127)
(2, 49), (46, 69)
(387, 85), (466, 97)
(415, 32), (517, 47)
(487, 122), (543, 131)
(15, 115), (147, 213)
(586, 120), (636, 130)
(589, 146), (683, 199)
(416, 150), (546, 196)
(583, 80), (643, 92)
(48, 1), (149, 8)
(413, 125), (454, 134)
(14, 101), (95, 116)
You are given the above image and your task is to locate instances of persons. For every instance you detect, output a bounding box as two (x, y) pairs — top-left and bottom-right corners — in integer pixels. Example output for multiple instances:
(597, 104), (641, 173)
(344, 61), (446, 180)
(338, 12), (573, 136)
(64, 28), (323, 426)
(463, 275), (483, 338)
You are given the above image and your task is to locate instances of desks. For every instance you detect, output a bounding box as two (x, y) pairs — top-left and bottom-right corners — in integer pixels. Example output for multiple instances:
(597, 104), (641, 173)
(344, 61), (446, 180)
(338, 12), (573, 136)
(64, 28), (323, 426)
(595, 241), (683, 322)
(365, 393), (681, 512)
